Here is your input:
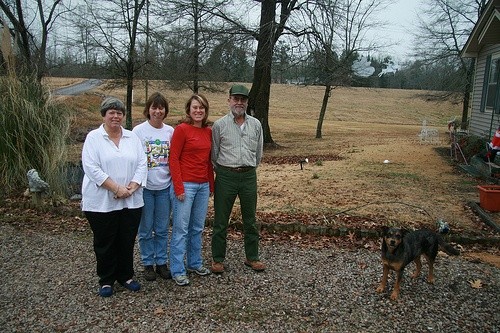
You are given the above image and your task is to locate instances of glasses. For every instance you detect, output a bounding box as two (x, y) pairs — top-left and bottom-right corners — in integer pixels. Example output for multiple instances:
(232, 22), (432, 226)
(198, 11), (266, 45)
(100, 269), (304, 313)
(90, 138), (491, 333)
(231, 97), (248, 103)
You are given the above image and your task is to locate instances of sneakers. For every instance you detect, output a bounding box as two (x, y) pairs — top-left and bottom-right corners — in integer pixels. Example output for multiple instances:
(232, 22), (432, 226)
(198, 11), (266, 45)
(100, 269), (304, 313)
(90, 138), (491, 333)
(187, 265), (210, 275)
(173, 274), (189, 285)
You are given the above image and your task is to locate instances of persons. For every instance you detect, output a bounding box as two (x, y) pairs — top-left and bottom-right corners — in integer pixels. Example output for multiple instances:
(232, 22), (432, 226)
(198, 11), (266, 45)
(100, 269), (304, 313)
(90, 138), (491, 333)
(169, 93), (214, 286)
(210, 85), (266, 273)
(81, 97), (148, 297)
(131, 92), (175, 281)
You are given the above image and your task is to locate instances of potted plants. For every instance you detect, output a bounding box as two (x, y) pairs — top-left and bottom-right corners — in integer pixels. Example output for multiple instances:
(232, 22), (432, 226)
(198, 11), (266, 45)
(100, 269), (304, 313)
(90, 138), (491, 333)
(458, 133), (490, 158)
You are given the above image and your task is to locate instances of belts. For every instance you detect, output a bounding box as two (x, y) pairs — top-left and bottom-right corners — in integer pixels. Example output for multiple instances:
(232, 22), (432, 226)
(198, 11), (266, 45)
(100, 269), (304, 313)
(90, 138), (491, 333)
(233, 166), (250, 172)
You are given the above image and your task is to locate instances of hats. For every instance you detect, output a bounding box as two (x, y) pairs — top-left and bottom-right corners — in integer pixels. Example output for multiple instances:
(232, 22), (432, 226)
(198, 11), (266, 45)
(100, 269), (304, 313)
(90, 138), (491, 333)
(229, 85), (249, 98)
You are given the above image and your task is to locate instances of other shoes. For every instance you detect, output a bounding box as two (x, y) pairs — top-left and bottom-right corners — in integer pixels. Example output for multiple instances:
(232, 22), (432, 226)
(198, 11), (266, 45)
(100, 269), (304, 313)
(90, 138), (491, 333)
(99, 284), (113, 297)
(212, 262), (224, 272)
(118, 279), (140, 290)
(245, 259), (265, 270)
(144, 265), (155, 280)
(156, 263), (171, 278)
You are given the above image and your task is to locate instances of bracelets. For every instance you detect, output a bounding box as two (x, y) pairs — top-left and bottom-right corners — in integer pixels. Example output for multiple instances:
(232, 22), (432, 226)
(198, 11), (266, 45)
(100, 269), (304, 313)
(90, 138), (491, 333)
(114, 184), (119, 195)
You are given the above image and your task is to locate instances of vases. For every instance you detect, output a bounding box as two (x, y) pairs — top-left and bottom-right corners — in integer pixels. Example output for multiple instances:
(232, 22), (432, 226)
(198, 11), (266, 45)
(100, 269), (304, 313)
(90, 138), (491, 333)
(476, 184), (500, 211)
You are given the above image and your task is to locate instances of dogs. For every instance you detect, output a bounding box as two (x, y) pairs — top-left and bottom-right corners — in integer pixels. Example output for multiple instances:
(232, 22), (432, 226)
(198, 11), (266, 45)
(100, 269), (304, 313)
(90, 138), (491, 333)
(376, 226), (438, 300)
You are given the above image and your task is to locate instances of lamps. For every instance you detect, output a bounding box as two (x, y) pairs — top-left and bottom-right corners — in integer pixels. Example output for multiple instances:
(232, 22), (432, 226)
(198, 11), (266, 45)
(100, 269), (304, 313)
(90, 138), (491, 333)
(299, 157), (310, 169)
